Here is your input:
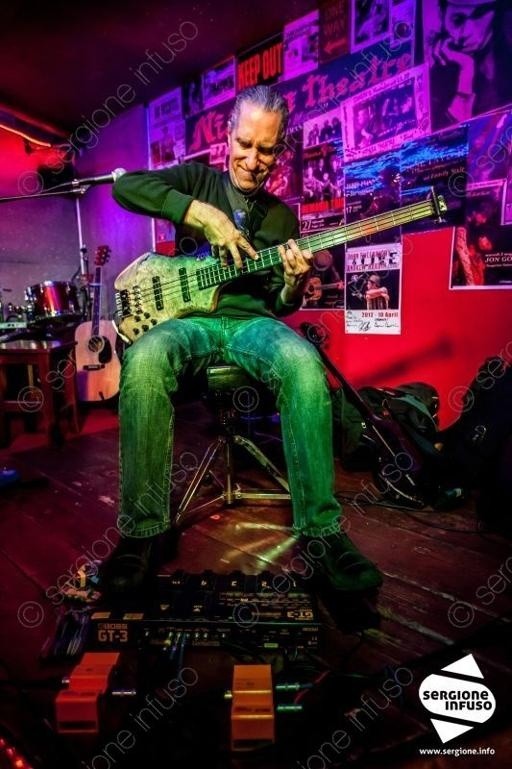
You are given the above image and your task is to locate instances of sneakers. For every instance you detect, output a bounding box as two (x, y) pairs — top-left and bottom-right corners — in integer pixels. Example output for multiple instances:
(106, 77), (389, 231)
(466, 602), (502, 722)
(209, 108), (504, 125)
(299, 529), (384, 591)
(97, 526), (179, 597)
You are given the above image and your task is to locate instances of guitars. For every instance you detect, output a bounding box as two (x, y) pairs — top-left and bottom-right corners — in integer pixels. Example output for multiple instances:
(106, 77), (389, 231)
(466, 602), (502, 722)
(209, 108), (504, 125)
(304, 277), (344, 302)
(66, 244), (123, 402)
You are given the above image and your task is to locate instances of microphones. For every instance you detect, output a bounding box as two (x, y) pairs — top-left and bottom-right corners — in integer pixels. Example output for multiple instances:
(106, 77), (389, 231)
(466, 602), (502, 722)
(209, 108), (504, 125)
(68, 168), (127, 186)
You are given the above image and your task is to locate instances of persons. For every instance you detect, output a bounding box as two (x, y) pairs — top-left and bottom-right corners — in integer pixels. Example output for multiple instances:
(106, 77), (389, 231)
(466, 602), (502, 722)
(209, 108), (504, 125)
(303, 155), (344, 202)
(430, 0), (512, 131)
(365, 275), (390, 310)
(452, 201), (493, 285)
(360, 97), (402, 144)
(308, 116), (340, 146)
(101, 86), (384, 595)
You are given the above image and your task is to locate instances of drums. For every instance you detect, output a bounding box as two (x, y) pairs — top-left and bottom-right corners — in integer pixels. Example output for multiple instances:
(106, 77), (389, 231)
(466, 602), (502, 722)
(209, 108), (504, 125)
(24, 279), (84, 332)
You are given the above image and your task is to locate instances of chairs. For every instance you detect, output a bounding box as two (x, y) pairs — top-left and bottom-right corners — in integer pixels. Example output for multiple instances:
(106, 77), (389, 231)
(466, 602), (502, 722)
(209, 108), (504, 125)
(115, 335), (310, 546)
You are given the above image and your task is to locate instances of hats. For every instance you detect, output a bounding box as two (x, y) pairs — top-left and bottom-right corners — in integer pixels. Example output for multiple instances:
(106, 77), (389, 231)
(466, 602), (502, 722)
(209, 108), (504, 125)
(312, 249), (333, 271)
(367, 275), (380, 286)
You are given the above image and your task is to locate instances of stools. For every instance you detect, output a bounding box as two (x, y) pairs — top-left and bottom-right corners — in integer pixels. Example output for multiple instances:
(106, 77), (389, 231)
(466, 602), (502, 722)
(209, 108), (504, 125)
(0, 339), (85, 448)
